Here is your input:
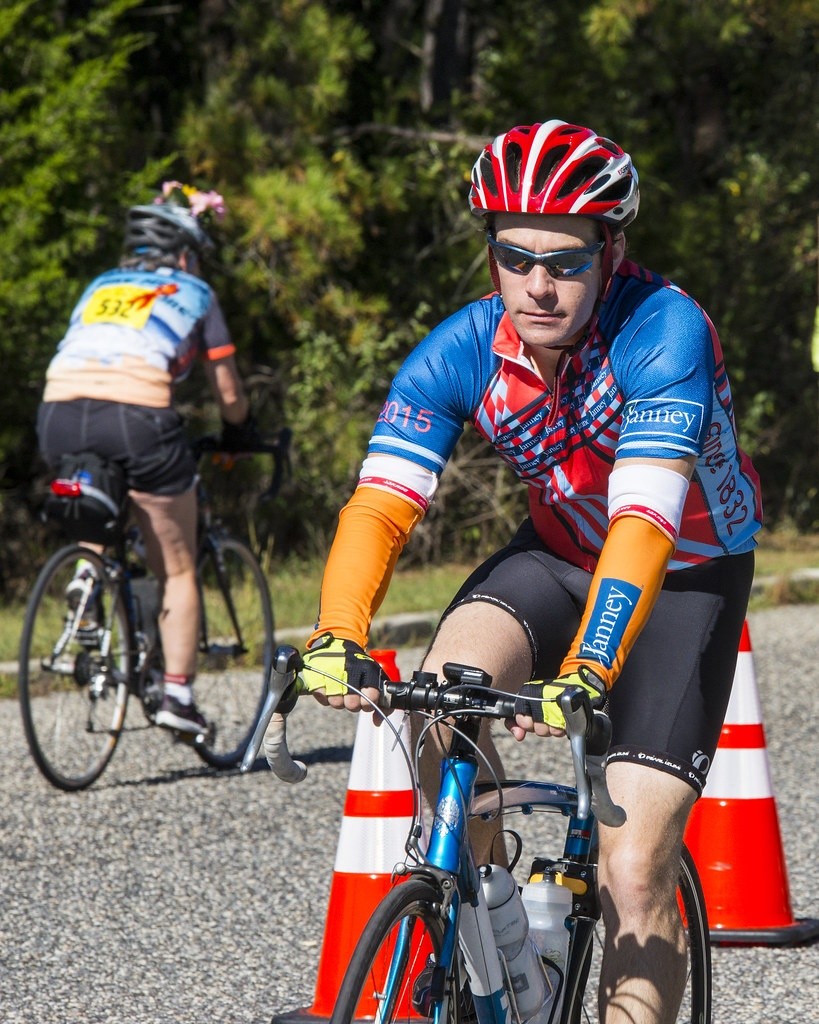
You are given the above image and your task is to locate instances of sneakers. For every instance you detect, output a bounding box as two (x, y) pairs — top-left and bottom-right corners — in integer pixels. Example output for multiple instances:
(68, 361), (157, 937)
(63, 570), (105, 650)
(155, 695), (209, 736)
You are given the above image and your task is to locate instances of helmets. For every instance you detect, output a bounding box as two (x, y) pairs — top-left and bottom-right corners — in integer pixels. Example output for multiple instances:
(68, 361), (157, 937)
(467, 119), (641, 232)
(122, 206), (215, 266)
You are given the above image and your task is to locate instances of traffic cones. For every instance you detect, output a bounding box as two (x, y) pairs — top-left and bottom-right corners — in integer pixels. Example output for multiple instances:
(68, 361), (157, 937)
(268, 648), (437, 1023)
(675, 618), (819, 949)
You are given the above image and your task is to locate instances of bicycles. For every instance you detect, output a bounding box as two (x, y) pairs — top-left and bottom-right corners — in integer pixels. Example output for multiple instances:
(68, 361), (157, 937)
(271, 633), (714, 1024)
(15, 425), (287, 795)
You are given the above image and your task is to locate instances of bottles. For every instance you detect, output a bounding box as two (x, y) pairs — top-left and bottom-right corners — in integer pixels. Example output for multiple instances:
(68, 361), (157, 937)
(509, 867), (573, 1024)
(135, 625), (151, 670)
(479, 862), (544, 1021)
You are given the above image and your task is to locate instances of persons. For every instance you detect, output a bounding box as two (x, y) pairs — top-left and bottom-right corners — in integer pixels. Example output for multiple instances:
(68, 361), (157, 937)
(305, 120), (765, 1024)
(40, 207), (248, 739)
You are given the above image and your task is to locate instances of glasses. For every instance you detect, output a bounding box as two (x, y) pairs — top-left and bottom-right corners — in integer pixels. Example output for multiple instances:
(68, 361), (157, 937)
(486, 231), (606, 278)
